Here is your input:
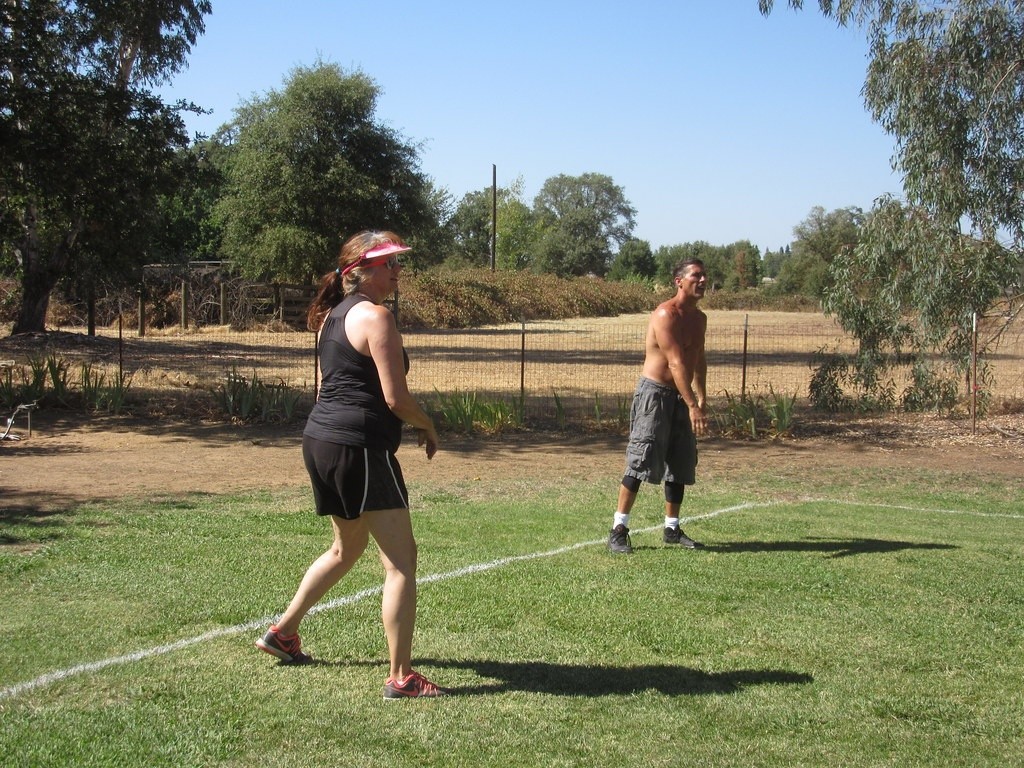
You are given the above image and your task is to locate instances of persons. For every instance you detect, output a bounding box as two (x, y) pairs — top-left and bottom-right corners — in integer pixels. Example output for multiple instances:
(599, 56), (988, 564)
(607, 258), (708, 556)
(257, 233), (447, 701)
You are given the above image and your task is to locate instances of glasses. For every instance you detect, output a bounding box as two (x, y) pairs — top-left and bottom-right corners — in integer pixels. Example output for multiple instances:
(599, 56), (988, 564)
(363, 255), (399, 270)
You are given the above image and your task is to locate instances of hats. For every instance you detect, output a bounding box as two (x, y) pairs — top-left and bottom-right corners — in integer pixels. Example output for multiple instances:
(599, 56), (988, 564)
(341, 241), (413, 276)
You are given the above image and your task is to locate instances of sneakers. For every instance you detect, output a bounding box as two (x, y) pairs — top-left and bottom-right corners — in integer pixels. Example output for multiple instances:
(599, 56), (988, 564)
(384, 671), (447, 700)
(608, 524), (634, 555)
(662, 524), (704, 548)
(256, 627), (312, 665)
